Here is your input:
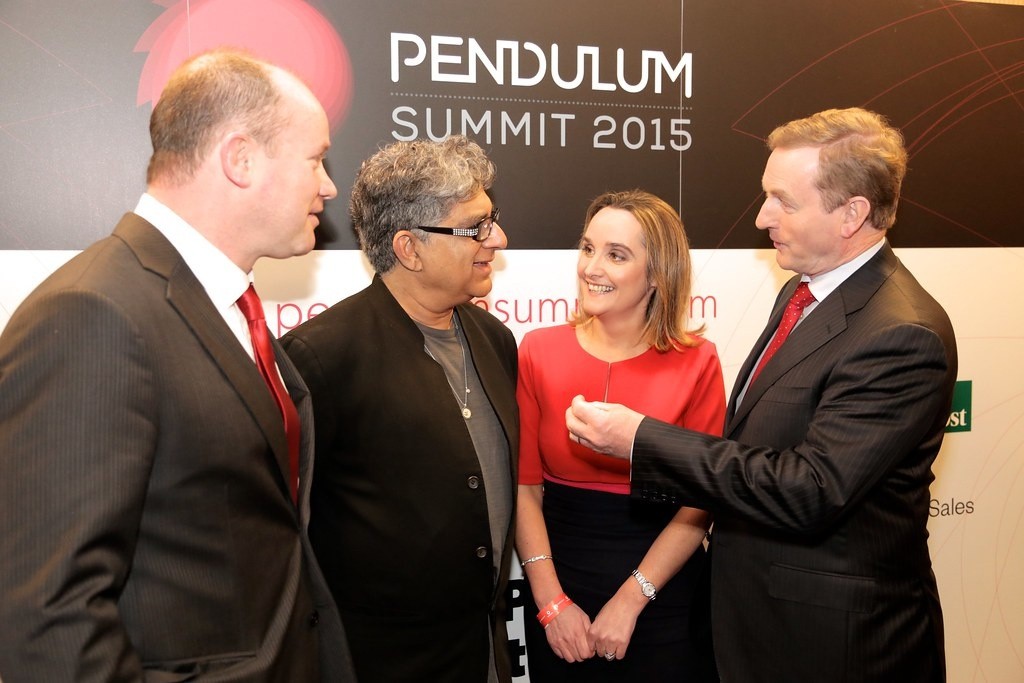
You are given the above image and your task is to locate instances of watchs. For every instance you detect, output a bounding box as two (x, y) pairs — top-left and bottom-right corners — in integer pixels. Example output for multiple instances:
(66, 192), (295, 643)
(632, 569), (657, 602)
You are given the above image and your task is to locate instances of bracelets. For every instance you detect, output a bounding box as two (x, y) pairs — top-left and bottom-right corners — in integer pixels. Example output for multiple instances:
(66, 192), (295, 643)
(537, 592), (575, 629)
(521, 555), (553, 567)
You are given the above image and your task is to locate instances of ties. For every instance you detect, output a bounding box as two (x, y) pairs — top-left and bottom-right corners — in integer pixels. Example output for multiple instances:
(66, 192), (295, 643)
(234, 282), (299, 507)
(746, 282), (816, 395)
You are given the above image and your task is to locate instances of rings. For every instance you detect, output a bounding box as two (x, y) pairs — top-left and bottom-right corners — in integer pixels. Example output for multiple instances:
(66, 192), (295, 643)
(605, 652), (615, 661)
(576, 437), (582, 446)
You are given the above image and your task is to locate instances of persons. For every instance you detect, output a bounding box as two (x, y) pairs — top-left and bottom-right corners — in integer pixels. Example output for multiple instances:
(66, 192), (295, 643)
(0, 53), (357, 683)
(564, 107), (958, 683)
(277, 136), (518, 683)
(515, 192), (726, 683)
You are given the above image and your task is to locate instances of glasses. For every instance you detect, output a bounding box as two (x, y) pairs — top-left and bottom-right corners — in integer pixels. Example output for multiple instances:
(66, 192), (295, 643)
(418, 207), (501, 242)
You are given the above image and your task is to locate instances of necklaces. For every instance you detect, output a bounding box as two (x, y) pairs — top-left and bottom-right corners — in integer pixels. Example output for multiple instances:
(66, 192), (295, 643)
(416, 316), (475, 422)
